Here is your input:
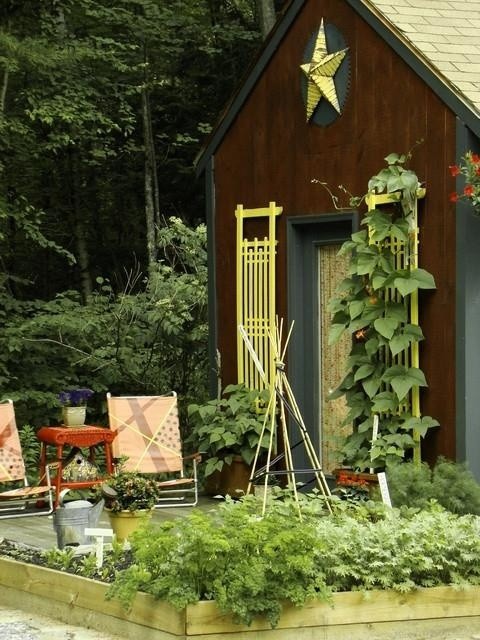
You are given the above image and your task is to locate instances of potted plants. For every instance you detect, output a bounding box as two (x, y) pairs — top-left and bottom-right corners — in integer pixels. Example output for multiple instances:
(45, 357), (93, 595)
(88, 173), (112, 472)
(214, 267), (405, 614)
(89, 455), (159, 545)
(184, 382), (281, 499)
(310, 135), (442, 487)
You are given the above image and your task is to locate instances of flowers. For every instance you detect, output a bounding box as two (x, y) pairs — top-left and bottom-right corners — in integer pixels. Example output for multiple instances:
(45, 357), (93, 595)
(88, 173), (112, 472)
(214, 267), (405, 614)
(56, 388), (96, 407)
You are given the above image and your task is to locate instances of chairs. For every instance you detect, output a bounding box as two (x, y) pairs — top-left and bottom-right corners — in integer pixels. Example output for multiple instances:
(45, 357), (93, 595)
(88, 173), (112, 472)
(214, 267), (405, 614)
(0, 391), (199, 520)
